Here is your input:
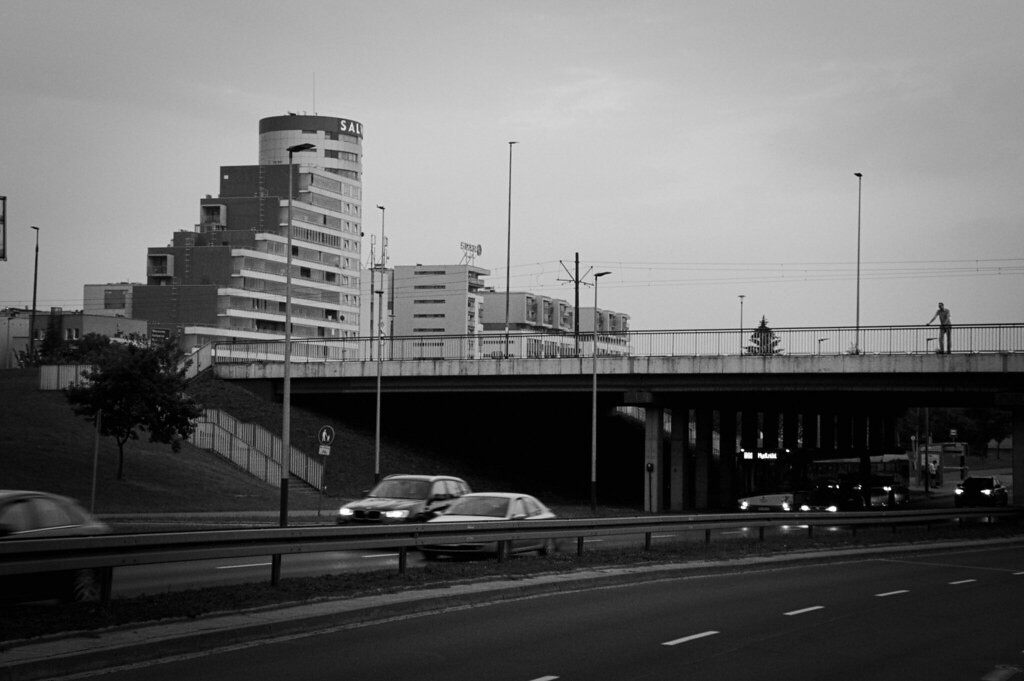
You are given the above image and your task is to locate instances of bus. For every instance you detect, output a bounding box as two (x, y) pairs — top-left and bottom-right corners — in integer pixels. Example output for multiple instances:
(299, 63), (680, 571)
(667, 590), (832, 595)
(738, 447), (872, 512)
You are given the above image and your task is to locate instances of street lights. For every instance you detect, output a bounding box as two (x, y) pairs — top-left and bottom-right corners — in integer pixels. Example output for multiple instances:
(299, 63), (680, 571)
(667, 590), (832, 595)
(505, 141), (517, 359)
(738, 294), (745, 356)
(278, 143), (316, 528)
(925, 338), (940, 492)
(818, 338), (829, 356)
(374, 203), (387, 491)
(854, 171), (863, 355)
(590, 272), (612, 512)
(29, 224), (38, 370)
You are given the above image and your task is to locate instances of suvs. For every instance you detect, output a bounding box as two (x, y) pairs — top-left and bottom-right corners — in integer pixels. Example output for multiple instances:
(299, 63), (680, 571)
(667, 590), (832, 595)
(336, 473), (472, 527)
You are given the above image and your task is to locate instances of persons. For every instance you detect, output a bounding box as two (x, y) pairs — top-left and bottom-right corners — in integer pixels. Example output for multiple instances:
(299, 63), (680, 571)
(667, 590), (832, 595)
(926, 303), (951, 354)
(929, 460), (937, 488)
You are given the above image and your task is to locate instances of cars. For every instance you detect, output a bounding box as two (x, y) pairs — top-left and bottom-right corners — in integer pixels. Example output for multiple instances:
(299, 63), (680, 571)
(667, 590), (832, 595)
(872, 486), (911, 509)
(418, 492), (557, 562)
(0, 488), (107, 607)
(799, 480), (872, 512)
(952, 475), (1009, 509)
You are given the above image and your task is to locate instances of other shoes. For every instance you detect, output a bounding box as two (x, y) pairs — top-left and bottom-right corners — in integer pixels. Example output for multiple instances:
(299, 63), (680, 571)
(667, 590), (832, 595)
(931, 486), (938, 488)
(936, 350), (951, 354)
